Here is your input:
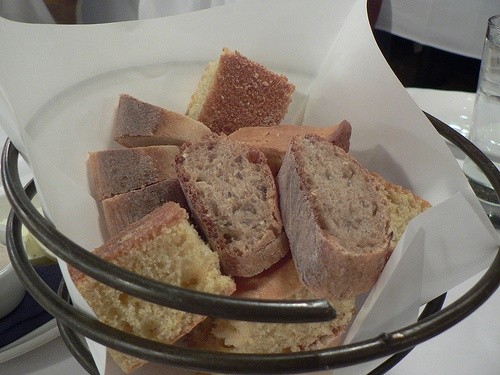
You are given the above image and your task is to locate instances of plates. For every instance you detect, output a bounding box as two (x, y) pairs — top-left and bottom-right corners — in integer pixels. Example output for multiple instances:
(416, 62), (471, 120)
(404, 88), (500, 218)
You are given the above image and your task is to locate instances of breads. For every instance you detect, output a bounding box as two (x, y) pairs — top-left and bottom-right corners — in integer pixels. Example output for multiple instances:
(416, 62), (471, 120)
(67, 47), (433, 374)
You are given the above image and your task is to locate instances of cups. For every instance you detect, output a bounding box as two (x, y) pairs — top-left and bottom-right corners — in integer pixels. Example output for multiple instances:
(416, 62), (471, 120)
(469, 14), (500, 163)
